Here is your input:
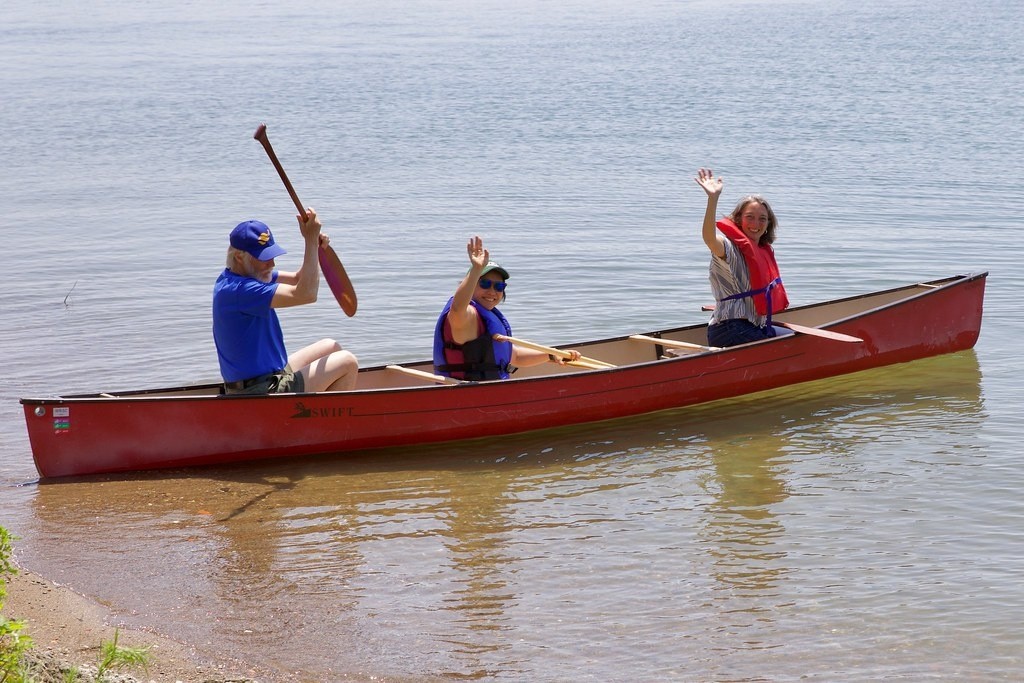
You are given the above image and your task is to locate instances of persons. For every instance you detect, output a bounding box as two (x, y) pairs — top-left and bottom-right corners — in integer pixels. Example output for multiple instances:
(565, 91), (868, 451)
(693, 166), (795, 349)
(432, 236), (582, 381)
(212, 208), (360, 395)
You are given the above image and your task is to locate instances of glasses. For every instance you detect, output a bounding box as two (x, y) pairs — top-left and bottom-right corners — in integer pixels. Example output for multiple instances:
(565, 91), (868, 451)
(478, 277), (508, 292)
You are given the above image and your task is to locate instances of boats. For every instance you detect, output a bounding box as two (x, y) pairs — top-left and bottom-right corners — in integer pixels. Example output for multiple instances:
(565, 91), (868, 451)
(15, 264), (991, 479)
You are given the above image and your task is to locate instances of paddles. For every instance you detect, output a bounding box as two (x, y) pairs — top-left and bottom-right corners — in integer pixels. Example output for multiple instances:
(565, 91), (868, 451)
(701, 304), (864, 344)
(492, 333), (618, 368)
(252, 122), (358, 318)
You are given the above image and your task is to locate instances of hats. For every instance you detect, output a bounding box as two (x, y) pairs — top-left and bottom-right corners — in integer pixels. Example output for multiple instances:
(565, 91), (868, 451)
(230, 220), (287, 261)
(467, 259), (509, 280)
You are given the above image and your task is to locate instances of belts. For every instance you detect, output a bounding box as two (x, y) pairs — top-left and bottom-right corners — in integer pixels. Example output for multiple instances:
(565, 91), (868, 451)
(224, 372), (272, 390)
